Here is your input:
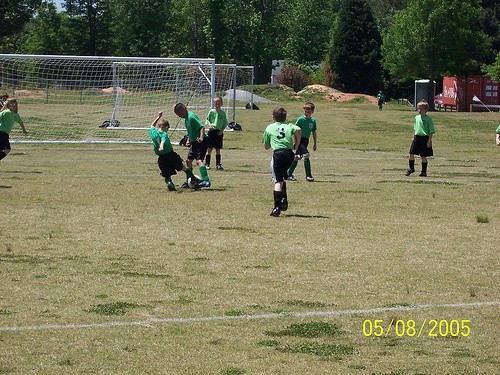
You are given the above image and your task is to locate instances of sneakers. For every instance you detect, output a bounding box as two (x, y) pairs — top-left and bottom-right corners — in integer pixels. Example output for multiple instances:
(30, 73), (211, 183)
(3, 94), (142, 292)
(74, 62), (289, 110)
(286, 175), (298, 181)
(419, 170), (427, 176)
(167, 182), (177, 192)
(270, 207), (280, 216)
(191, 180), (201, 186)
(196, 180), (211, 187)
(405, 168), (415, 176)
(281, 199), (288, 210)
(205, 164), (210, 170)
(216, 164), (223, 170)
(306, 176), (314, 182)
(181, 183), (189, 188)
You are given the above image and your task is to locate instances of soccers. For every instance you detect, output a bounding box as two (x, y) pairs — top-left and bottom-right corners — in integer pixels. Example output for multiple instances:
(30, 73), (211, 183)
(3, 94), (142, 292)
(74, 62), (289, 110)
(187, 175), (201, 188)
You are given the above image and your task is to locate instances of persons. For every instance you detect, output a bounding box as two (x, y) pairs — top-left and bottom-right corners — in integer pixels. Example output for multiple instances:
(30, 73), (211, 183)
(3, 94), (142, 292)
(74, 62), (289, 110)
(0, 92), (29, 160)
(149, 111), (201, 191)
(204, 96), (228, 169)
(404, 101), (435, 177)
(495, 125), (500, 145)
(286, 102), (317, 181)
(173, 102), (211, 188)
(262, 106), (302, 217)
(377, 89), (385, 111)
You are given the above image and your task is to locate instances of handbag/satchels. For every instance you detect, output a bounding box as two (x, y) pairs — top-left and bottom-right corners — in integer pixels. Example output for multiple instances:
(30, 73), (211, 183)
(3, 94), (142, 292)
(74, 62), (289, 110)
(180, 134), (188, 146)
(228, 122), (242, 131)
(246, 102), (259, 110)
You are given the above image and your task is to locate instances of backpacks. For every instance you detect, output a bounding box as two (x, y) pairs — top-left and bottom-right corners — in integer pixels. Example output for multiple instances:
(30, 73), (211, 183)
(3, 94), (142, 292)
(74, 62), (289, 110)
(99, 120), (120, 129)
